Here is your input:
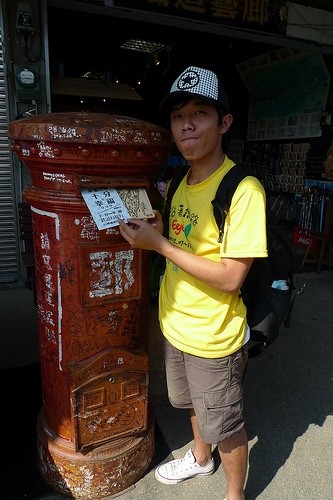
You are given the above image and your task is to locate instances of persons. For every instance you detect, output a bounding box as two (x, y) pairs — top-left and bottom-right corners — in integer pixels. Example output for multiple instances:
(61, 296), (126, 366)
(118, 66), (268, 500)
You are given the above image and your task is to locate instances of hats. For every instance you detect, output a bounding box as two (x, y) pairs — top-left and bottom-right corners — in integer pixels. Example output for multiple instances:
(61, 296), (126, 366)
(160, 66), (229, 113)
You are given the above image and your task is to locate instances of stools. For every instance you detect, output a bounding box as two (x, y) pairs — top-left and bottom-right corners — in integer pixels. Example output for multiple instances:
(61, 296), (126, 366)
(299, 232), (332, 275)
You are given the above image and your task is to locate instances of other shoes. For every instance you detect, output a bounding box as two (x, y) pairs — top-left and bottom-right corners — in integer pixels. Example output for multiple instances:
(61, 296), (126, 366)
(154, 448), (214, 485)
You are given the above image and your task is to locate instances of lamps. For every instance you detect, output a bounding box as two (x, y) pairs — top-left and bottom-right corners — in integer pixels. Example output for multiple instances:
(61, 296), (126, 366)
(154, 60), (160, 65)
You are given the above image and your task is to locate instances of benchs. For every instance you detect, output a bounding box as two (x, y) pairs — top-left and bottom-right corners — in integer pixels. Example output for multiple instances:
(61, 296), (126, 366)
(17, 202), (34, 290)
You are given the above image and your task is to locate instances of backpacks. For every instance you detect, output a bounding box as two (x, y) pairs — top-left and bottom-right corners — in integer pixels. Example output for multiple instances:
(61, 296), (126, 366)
(162, 165), (306, 359)
